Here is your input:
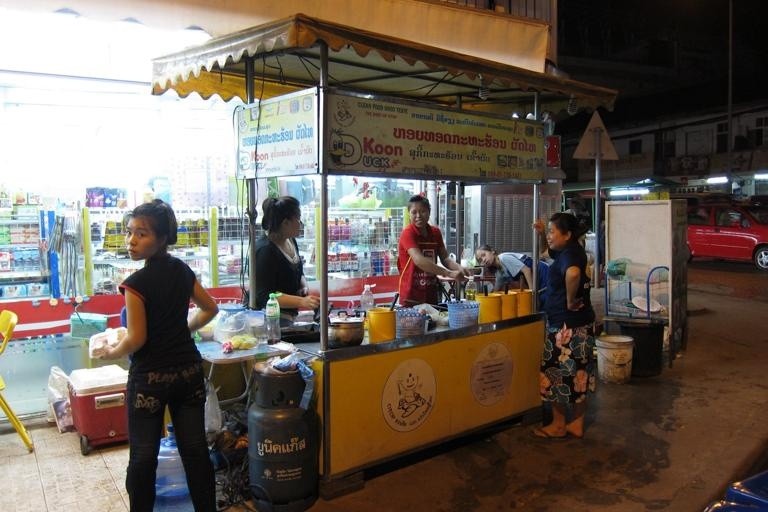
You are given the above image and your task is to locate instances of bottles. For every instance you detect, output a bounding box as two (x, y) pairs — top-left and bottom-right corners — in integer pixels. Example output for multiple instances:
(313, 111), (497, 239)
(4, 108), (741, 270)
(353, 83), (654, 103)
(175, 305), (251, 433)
(440, 194), (464, 236)
(265, 292), (282, 345)
(360, 283), (377, 330)
(327, 213), (404, 276)
(174, 216), (260, 247)
(465, 275), (477, 301)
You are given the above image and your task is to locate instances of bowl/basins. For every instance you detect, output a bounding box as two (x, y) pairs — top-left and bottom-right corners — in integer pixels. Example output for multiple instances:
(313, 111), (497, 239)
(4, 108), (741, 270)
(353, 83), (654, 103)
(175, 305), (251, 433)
(197, 331), (214, 340)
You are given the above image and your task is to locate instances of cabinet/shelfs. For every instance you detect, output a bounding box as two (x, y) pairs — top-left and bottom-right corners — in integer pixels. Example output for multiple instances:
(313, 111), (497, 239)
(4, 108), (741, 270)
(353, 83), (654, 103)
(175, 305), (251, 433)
(328, 220), (402, 278)
(90, 215), (209, 294)
(216, 217), (317, 287)
(0, 219), (49, 302)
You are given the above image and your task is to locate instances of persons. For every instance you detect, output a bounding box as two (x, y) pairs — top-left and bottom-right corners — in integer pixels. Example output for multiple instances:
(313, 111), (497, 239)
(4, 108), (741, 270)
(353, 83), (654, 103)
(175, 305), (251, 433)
(533, 212), (595, 438)
(100, 199), (220, 512)
(397, 196), (465, 309)
(476, 245), (552, 312)
(244, 196), (321, 328)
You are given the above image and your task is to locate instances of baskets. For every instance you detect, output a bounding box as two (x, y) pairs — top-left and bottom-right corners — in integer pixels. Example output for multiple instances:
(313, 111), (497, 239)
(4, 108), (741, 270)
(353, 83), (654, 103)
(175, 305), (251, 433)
(396, 307), (425, 339)
(447, 300), (479, 330)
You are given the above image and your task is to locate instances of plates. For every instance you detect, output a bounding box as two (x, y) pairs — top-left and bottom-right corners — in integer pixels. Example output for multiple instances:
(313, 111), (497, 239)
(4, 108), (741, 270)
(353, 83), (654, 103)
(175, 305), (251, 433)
(631, 296), (661, 313)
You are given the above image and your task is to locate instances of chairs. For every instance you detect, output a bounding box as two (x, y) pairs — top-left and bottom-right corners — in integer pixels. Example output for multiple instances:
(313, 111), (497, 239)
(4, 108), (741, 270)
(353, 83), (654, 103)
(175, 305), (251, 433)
(0, 310), (34, 453)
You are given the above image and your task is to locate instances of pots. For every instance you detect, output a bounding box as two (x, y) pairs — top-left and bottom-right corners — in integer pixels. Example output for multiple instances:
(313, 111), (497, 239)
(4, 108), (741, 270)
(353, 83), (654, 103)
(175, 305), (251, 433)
(327, 310), (366, 349)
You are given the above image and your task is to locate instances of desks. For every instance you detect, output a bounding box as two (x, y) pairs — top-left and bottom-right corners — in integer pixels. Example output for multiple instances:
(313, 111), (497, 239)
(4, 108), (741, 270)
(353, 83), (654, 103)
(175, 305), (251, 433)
(195, 336), (292, 421)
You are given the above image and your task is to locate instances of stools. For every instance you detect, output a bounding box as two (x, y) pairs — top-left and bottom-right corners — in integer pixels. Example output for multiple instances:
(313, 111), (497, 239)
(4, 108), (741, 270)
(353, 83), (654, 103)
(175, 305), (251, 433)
(728, 460), (768, 506)
(701, 498), (767, 512)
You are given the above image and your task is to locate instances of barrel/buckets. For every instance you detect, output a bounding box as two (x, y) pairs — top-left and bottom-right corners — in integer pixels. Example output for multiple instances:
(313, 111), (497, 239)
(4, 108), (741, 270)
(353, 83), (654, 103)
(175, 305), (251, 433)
(595, 336), (634, 384)
(618, 323), (666, 378)
(152, 422), (198, 512)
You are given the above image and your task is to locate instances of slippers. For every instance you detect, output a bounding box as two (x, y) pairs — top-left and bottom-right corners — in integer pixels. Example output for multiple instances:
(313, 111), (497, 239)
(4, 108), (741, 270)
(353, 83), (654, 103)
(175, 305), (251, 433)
(530, 427), (569, 442)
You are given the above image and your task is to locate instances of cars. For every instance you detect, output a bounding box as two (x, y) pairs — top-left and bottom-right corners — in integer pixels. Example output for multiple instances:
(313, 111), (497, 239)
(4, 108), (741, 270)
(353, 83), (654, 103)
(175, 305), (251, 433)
(686, 200), (768, 270)
